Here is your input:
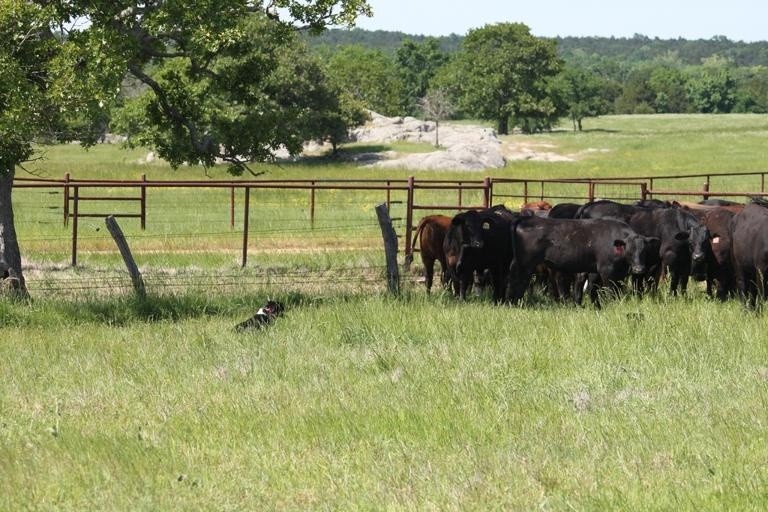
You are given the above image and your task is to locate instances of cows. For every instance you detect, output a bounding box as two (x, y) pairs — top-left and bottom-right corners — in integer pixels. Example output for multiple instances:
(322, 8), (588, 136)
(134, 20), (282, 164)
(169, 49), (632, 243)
(404, 196), (768, 317)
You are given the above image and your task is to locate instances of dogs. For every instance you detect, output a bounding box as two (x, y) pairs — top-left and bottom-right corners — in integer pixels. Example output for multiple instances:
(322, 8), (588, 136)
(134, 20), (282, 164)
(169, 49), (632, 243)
(230, 301), (285, 334)
